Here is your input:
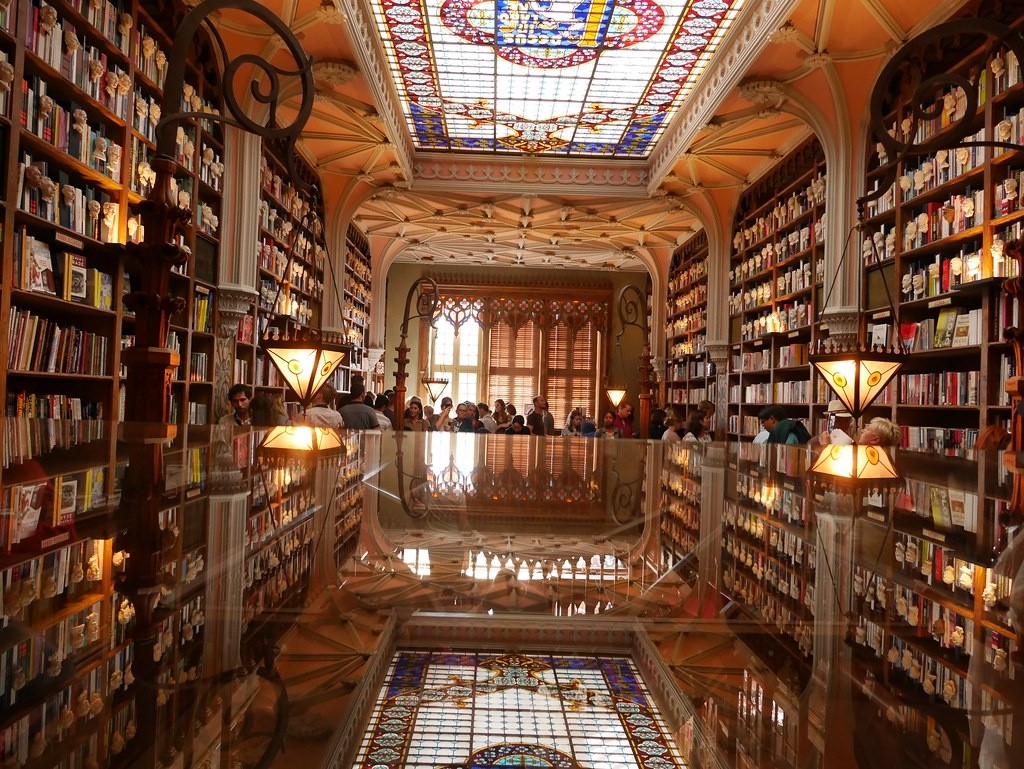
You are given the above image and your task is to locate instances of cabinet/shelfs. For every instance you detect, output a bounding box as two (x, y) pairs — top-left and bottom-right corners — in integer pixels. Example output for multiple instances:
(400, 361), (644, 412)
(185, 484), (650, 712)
(652, 442), (1024, 769)
(342, 221), (373, 331)
(725, 134), (827, 445)
(0, 0), (227, 421)
(0, 418), (365, 769)
(226, 116), (328, 414)
(665, 226), (716, 437)
(852, 15), (1024, 577)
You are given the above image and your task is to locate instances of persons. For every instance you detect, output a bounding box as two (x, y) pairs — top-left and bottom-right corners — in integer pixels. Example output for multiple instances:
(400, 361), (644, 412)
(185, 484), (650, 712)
(751, 399), (851, 444)
(218, 383), (251, 424)
(975, 424), (1010, 450)
(248, 397), (297, 425)
(299, 374), (395, 428)
(651, 400), (715, 440)
(858, 417), (899, 445)
(561, 401), (633, 438)
(404, 396), (554, 436)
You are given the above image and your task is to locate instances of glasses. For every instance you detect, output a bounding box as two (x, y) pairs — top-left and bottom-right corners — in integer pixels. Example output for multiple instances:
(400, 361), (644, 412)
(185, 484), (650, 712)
(759, 418), (768, 425)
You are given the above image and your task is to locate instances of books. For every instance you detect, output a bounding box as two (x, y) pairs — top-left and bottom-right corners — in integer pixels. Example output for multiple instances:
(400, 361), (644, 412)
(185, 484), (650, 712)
(0, 424), (1000, 769)
(645, 49), (1024, 466)
(0, 0), (372, 423)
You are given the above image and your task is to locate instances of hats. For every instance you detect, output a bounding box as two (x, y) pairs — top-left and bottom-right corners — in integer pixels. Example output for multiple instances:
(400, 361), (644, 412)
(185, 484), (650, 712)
(823, 400), (852, 417)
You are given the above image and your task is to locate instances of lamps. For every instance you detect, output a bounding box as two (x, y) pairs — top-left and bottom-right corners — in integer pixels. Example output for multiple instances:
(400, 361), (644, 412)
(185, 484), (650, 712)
(422, 376), (450, 403)
(604, 387), (629, 409)
(811, 203), (909, 431)
(806, 446), (907, 624)
(261, 210), (354, 414)
(255, 425), (347, 600)
